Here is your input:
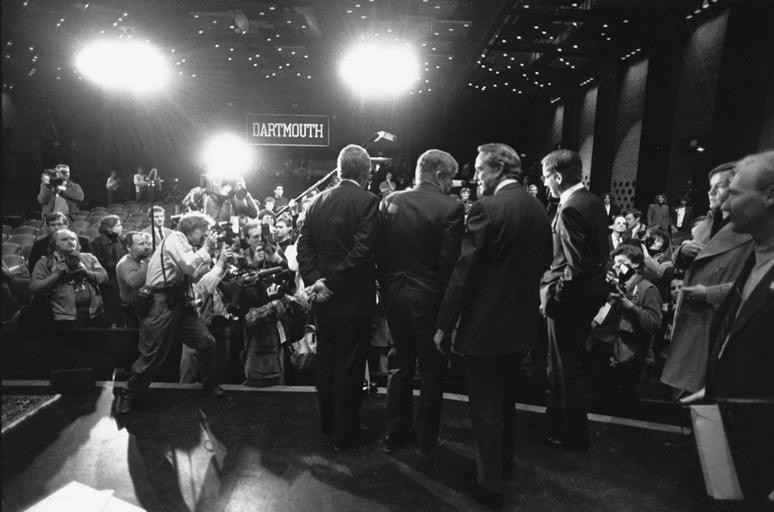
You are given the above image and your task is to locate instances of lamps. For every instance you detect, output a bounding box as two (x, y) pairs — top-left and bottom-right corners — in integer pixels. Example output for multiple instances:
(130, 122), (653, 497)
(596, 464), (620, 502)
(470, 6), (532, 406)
(47, 366), (96, 396)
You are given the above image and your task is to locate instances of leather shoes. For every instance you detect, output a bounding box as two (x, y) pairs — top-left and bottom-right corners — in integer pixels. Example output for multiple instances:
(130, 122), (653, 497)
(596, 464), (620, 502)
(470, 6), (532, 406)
(472, 456), (513, 479)
(117, 393), (138, 414)
(201, 383), (225, 397)
(462, 470), (504, 506)
(529, 413), (591, 452)
(319, 426), (448, 459)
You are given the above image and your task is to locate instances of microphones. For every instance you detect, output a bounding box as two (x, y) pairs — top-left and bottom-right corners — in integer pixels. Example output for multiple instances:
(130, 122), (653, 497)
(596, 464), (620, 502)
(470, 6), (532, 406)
(377, 130), (397, 142)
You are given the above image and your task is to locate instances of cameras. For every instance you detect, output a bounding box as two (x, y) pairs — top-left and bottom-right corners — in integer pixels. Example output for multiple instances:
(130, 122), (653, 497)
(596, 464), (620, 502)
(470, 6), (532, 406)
(205, 222), (239, 247)
(606, 263), (634, 292)
(64, 253), (80, 270)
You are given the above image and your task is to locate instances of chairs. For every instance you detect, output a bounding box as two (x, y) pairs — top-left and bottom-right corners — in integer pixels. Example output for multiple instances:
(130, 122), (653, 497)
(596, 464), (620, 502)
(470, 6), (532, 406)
(1, 195), (186, 343)
